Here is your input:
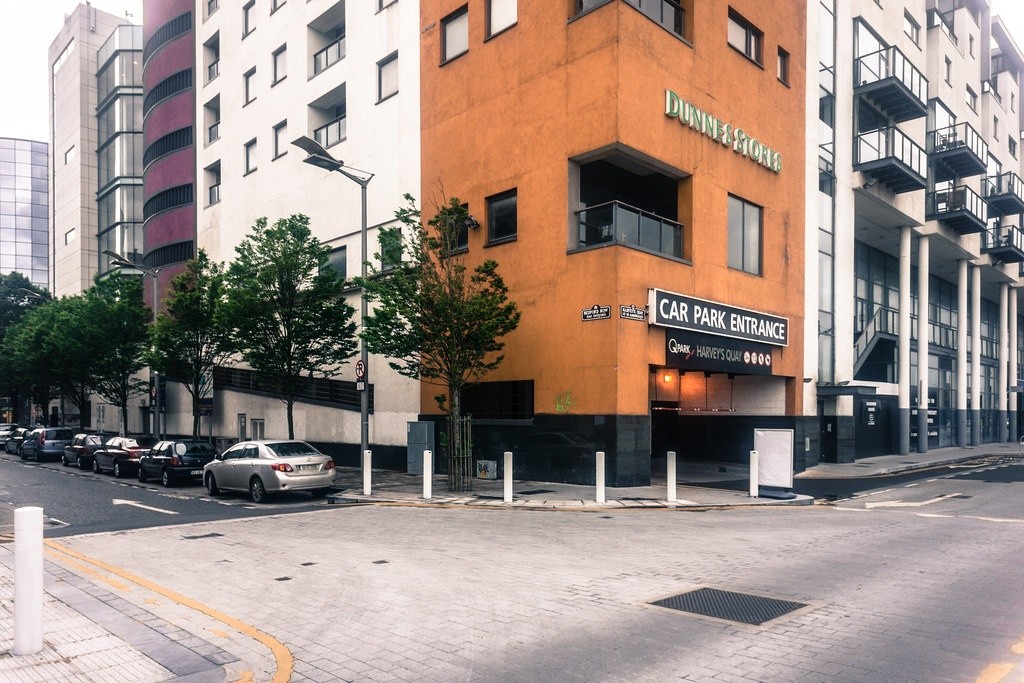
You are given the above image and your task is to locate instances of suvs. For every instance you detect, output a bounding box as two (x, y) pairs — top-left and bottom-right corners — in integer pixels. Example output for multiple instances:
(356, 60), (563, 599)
(20, 427), (74, 462)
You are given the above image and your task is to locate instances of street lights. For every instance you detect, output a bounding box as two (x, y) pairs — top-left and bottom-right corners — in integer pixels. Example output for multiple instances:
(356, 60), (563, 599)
(102, 249), (162, 438)
(290, 136), (377, 488)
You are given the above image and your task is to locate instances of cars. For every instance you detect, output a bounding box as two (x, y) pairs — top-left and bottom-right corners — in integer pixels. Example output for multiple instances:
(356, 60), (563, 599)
(137, 439), (221, 488)
(5, 428), (35, 456)
(203, 439), (337, 504)
(0, 423), (22, 449)
(62, 433), (114, 470)
(92, 435), (160, 477)
